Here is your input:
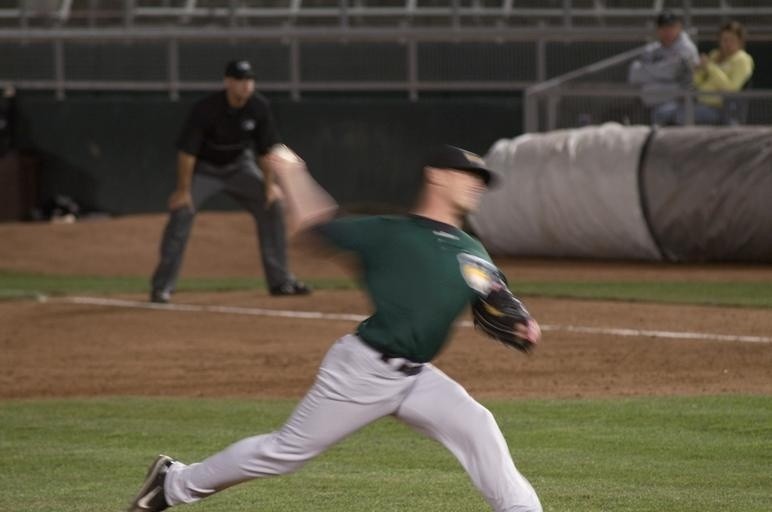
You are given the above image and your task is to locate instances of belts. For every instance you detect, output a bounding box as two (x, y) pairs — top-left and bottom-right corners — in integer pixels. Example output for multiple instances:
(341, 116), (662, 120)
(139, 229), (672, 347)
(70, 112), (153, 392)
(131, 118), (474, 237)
(381, 354), (420, 375)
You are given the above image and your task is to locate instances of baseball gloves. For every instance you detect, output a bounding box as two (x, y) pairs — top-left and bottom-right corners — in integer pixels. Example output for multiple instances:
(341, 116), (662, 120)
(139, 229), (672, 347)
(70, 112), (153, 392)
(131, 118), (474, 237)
(474, 285), (541, 351)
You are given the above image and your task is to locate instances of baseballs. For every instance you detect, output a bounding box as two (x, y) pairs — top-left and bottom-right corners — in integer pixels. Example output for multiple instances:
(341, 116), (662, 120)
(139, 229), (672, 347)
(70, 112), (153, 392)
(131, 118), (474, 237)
(271, 143), (298, 165)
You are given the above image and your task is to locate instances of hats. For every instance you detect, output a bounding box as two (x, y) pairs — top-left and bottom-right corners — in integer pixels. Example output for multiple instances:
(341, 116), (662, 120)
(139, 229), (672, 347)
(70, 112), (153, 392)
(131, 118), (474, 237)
(428, 144), (490, 184)
(225, 59), (257, 76)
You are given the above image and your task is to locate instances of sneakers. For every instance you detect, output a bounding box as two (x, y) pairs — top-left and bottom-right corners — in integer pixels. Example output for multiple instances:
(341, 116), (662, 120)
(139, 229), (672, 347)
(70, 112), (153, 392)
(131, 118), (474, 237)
(270, 278), (311, 295)
(152, 288), (170, 302)
(128, 454), (175, 512)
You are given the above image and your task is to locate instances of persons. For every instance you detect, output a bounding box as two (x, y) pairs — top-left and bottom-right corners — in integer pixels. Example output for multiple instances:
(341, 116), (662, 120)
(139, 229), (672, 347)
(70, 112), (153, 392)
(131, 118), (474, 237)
(130, 141), (544, 512)
(630, 13), (700, 125)
(695, 20), (752, 105)
(151, 57), (308, 301)
